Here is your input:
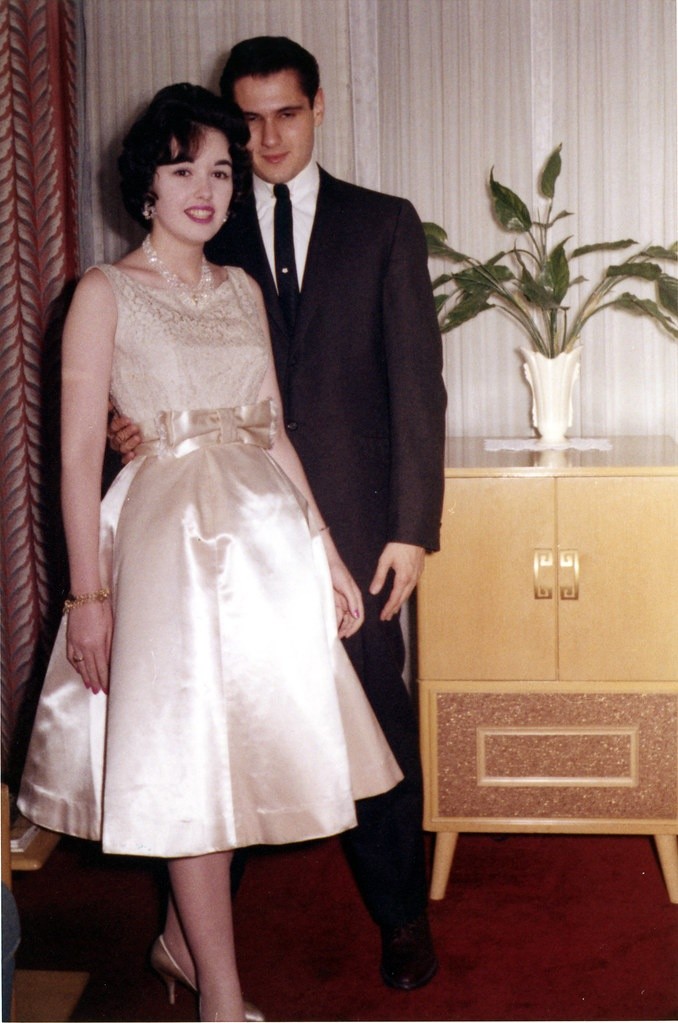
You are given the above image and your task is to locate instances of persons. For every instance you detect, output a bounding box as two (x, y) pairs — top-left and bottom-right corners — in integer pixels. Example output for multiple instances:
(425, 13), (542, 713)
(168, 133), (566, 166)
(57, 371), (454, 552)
(17, 83), (405, 1022)
(108, 36), (448, 991)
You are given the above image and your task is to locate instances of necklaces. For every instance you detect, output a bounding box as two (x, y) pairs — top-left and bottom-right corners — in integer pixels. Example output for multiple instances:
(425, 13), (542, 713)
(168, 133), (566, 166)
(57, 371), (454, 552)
(142, 234), (214, 312)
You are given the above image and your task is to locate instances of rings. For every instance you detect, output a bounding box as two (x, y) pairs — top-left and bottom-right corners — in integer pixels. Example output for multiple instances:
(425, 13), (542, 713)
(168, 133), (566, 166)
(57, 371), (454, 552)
(74, 656), (84, 662)
(345, 611), (350, 614)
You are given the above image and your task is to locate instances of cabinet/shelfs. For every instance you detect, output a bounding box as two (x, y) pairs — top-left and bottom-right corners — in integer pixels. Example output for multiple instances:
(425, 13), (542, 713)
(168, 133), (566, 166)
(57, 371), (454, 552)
(416, 438), (678, 903)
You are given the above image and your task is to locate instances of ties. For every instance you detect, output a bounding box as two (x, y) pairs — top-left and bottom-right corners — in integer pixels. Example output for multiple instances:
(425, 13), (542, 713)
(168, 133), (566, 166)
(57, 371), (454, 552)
(273, 182), (300, 337)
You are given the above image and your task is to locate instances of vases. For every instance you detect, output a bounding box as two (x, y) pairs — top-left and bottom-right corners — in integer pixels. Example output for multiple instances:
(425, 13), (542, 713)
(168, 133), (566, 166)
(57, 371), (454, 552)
(519, 346), (584, 443)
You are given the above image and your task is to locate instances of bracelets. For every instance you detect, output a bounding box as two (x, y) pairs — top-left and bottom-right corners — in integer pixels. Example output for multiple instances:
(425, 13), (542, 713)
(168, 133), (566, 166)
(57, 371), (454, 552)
(63, 588), (111, 608)
(320, 527), (330, 535)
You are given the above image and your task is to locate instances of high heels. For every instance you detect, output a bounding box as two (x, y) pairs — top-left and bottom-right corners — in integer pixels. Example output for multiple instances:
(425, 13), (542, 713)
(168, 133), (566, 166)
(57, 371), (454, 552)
(150, 935), (265, 1022)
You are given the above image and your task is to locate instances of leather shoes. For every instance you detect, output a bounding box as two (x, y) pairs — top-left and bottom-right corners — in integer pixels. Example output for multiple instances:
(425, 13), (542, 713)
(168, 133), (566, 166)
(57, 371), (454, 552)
(381, 908), (437, 990)
